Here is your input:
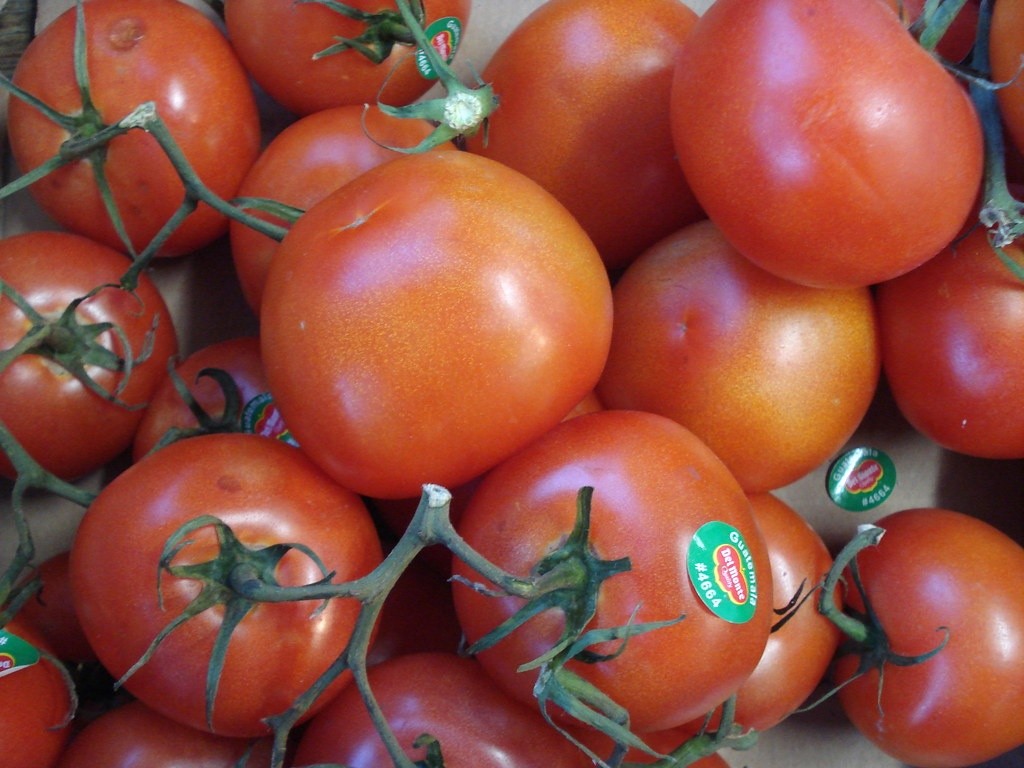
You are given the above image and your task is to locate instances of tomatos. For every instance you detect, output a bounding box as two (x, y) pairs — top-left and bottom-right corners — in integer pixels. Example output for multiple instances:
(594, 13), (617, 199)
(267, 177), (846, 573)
(0, 0), (1024, 768)
